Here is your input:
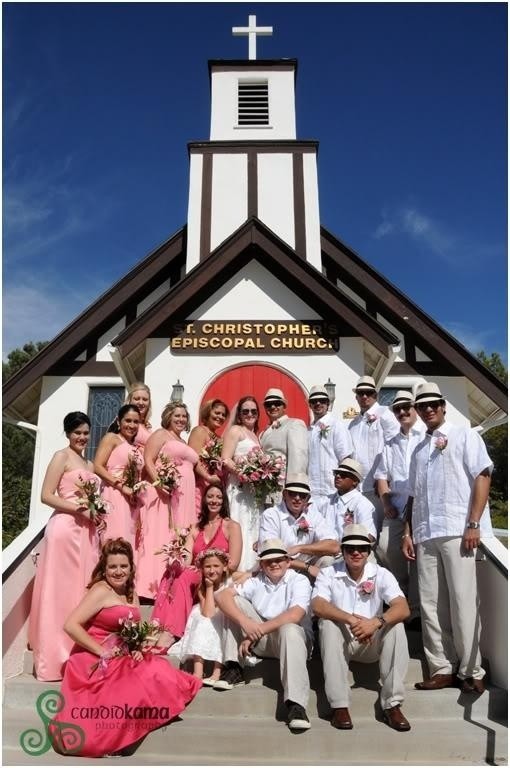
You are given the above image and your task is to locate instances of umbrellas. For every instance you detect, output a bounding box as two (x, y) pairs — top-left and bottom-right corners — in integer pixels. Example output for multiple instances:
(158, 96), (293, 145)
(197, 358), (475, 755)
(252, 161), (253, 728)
(383, 705), (411, 732)
(462, 677), (486, 694)
(331, 708), (354, 731)
(414, 672), (456, 690)
(202, 675), (216, 687)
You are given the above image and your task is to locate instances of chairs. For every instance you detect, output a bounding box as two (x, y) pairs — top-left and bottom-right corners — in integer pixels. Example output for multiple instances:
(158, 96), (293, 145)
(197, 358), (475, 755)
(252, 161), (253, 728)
(286, 703), (312, 731)
(213, 667), (245, 692)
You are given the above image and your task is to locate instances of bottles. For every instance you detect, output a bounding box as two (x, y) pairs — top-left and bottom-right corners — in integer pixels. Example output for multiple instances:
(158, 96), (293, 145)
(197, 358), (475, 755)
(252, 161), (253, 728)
(351, 375), (377, 391)
(332, 458), (366, 484)
(414, 382), (448, 404)
(388, 390), (414, 411)
(309, 385), (329, 400)
(263, 388), (288, 406)
(341, 523), (375, 547)
(256, 538), (288, 560)
(285, 472), (311, 495)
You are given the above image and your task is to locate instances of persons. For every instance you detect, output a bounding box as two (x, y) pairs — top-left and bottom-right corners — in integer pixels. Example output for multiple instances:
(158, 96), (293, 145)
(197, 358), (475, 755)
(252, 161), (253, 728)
(212, 538), (317, 729)
(136, 402), (198, 600)
(167, 549), (259, 687)
(258, 473), (340, 578)
(310, 524), (410, 731)
(47, 537), (202, 758)
(253, 459), (378, 564)
(28, 411), (107, 681)
(142, 483), (242, 655)
(347, 376), (400, 529)
(258, 388), (308, 508)
(374, 389), (426, 632)
(221, 396), (264, 572)
(188, 399), (227, 524)
(94, 404), (145, 555)
(401, 382), (494, 693)
(124, 383), (152, 448)
(307, 385), (354, 497)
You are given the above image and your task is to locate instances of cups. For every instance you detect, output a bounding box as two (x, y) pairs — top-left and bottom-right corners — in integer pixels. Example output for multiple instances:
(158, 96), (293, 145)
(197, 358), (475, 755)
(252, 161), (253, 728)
(288, 490), (307, 499)
(356, 389), (375, 397)
(310, 399), (327, 404)
(417, 401), (443, 411)
(332, 471), (350, 479)
(264, 401), (282, 407)
(242, 408), (258, 415)
(343, 545), (368, 554)
(394, 404), (414, 413)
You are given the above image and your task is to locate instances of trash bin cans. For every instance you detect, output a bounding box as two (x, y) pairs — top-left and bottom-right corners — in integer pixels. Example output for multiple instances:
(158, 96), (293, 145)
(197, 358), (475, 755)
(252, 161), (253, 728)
(466, 523), (480, 528)
(376, 616), (386, 630)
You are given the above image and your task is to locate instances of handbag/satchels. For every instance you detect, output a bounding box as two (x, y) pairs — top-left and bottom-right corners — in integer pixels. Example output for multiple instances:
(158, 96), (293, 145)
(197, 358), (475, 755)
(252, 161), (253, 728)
(303, 563), (311, 572)
(400, 534), (410, 540)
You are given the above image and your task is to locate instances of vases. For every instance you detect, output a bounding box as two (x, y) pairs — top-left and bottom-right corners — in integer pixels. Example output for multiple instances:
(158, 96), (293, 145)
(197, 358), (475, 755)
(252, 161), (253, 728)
(120, 451), (148, 492)
(356, 579), (375, 594)
(154, 525), (193, 569)
(73, 474), (114, 519)
(151, 451), (182, 492)
(317, 423), (330, 436)
(200, 433), (223, 471)
(113, 612), (164, 656)
(296, 517), (313, 536)
(434, 436), (448, 450)
(235, 445), (285, 498)
(343, 508), (354, 525)
(367, 414), (377, 425)
